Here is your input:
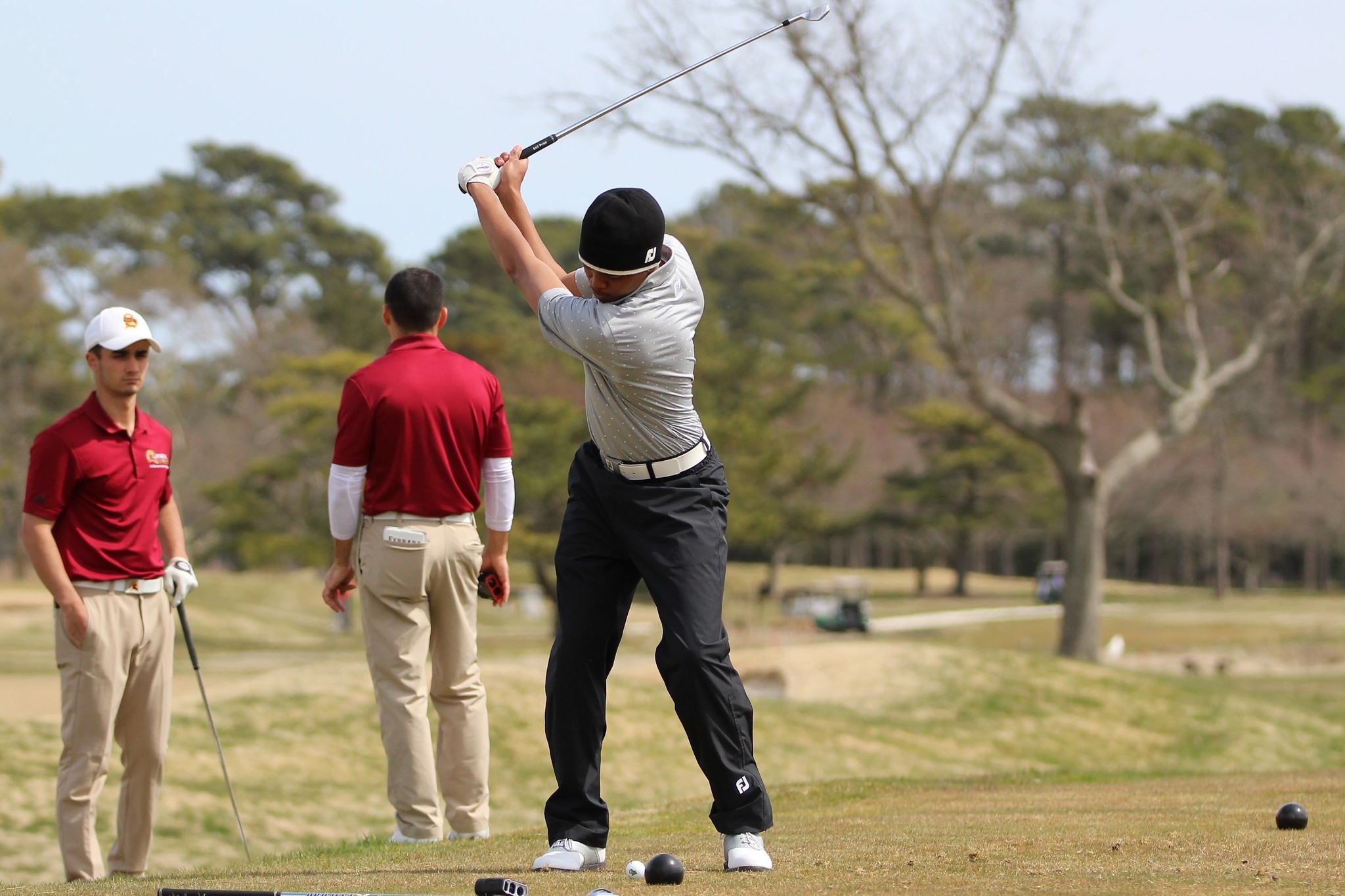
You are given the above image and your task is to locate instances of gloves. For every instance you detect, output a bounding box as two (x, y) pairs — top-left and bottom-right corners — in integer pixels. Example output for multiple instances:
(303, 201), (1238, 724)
(457, 157), (503, 197)
(164, 556), (198, 608)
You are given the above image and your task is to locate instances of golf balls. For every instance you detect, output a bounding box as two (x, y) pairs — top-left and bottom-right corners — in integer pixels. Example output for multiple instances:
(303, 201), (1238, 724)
(626, 861), (645, 879)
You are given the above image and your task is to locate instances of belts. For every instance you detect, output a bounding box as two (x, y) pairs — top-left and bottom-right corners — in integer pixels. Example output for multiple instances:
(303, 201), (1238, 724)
(70, 575), (163, 594)
(362, 510), (474, 524)
(600, 428), (710, 480)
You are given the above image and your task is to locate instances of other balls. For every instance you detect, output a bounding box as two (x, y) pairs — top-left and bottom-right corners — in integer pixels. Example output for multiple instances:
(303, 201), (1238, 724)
(1276, 802), (1308, 831)
(644, 853), (684, 886)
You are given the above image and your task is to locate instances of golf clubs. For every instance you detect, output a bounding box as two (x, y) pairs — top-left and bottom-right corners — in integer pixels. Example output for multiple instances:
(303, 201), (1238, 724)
(156, 886), (618, 896)
(171, 579), (251, 861)
(457, 3), (830, 195)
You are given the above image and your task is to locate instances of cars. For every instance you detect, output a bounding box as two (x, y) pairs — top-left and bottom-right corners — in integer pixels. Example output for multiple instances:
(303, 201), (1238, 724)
(1033, 559), (1070, 601)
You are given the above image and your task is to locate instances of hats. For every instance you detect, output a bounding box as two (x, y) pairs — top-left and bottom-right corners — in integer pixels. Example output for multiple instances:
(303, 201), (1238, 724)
(578, 188), (665, 275)
(84, 307), (162, 354)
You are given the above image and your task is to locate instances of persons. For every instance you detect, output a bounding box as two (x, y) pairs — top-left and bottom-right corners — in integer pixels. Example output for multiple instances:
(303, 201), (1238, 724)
(460, 143), (776, 870)
(23, 307), (200, 876)
(322, 268), (514, 843)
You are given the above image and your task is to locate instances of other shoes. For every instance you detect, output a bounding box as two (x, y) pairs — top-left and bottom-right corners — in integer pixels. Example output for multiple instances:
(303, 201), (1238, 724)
(388, 824), (444, 843)
(447, 827), (490, 840)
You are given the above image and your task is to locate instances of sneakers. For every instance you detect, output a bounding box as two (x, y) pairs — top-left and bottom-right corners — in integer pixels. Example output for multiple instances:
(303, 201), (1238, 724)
(724, 832), (772, 873)
(533, 838), (606, 871)
